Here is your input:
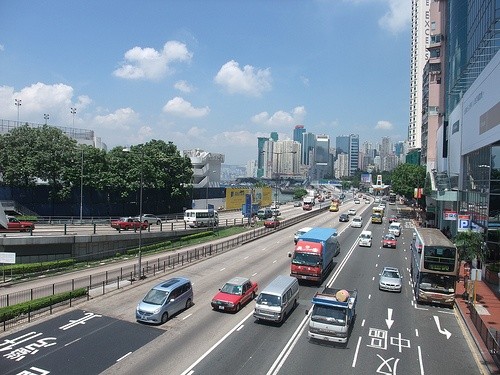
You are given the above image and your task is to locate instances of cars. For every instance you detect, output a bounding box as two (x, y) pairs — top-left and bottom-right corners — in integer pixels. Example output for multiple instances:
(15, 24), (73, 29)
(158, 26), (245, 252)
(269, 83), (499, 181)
(111, 217), (149, 231)
(382, 234), (397, 249)
(339, 190), (401, 236)
(294, 202), (302, 207)
(303, 188), (332, 206)
(210, 277), (258, 313)
(358, 230), (373, 247)
(334, 241), (340, 257)
(328, 204), (339, 212)
(303, 204), (312, 210)
(0, 215), (36, 233)
(134, 214), (162, 225)
(378, 266), (404, 293)
(257, 204), (281, 220)
(264, 217), (281, 228)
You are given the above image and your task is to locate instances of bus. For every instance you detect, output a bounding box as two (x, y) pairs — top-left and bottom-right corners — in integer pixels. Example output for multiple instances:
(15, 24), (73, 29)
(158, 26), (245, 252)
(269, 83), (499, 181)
(184, 209), (220, 228)
(410, 226), (460, 308)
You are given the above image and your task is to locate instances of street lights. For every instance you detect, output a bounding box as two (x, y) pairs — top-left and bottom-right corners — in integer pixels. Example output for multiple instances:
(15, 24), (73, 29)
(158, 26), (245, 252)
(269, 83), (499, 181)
(261, 151), (297, 229)
(122, 148), (145, 281)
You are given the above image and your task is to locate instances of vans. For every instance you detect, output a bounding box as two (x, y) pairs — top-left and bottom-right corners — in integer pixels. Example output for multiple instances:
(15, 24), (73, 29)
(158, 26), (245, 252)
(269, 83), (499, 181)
(253, 275), (299, 326)
(293, 227), (312, 245)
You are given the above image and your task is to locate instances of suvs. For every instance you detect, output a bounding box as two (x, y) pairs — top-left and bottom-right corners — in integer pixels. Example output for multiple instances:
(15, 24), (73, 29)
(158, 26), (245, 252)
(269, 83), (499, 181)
(135, 277), (193, 326)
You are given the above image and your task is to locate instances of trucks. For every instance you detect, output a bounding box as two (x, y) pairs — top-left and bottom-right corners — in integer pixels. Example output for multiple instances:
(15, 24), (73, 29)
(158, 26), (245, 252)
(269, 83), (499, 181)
(288, 227), (338, 287)
(304, 285), (358, 346)
